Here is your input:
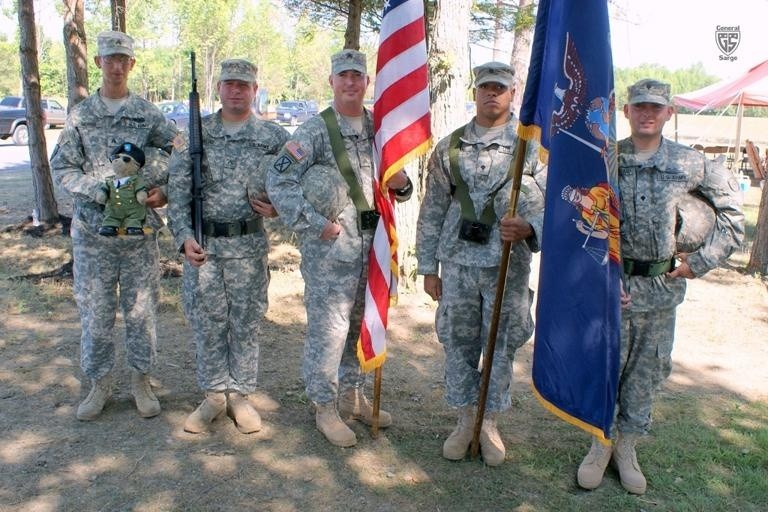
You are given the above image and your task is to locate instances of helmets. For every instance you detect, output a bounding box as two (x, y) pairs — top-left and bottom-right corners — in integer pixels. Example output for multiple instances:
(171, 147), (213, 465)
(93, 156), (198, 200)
(493, 174), (545, 222)
(247, 154), (278, 213)
(301, 163), (351, 222)
(138, 146), (171, 190)
(676, 193), (717, 253)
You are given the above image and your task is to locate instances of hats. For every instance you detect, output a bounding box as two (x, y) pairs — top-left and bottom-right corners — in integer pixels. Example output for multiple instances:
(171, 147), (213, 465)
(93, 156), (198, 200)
(474, 62), (516, 88)
(219, 59), (258, 82)
(331, 49), (367, 75)
(626, 79), (671, 106)
(97, 31), (134, 58)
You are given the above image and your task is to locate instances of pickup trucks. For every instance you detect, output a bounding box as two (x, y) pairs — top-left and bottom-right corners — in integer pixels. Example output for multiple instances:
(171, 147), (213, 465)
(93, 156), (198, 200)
(275, 99), (318, 126)
(0, 105), (51, 145)
(153, 101), (210, 130)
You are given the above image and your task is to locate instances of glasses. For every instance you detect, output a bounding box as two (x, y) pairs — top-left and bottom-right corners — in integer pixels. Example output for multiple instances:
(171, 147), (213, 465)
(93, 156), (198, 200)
(102, 55), (131, 65)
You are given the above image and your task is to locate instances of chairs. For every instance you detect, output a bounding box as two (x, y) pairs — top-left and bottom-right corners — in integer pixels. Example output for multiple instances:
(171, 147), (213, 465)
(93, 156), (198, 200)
(689, 140), (768, 181)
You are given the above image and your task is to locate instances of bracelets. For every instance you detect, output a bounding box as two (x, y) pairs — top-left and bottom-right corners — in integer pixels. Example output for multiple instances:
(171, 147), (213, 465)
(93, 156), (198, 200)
(394, 172), (412, 203)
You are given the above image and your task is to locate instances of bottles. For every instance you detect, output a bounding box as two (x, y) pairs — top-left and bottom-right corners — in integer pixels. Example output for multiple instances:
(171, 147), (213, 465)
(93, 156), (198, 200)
(32, 209), (40, 226)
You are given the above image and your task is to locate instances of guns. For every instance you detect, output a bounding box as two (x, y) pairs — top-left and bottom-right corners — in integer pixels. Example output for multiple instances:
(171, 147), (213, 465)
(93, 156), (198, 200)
(190, 50), (204, 261)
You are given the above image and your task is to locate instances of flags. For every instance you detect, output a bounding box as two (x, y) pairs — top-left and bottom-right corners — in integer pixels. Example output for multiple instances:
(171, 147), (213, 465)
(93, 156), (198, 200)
(358, 1), (440, 376)
(517, 0), (622, 447)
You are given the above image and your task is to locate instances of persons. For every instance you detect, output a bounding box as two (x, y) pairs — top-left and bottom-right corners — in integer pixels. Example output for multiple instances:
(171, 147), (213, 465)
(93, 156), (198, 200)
(163, 59), (293, 437)
(415, 60), (546, 471)
(48, 28), (174, 416)
(267, 50), (413, 448)
(574, 79), (746, 496)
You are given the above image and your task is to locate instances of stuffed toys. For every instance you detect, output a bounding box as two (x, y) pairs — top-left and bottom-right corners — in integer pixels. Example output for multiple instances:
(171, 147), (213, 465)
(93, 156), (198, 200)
(93, 140), (150, 234)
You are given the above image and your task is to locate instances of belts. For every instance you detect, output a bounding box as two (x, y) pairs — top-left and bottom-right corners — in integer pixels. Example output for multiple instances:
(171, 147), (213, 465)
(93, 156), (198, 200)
(201, 216), (263, 237)
(621, 257), (675, 277)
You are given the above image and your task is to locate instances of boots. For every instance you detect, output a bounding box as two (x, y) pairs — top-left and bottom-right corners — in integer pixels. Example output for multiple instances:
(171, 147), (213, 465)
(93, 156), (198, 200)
(226, 390), (262, 434)
(337, 383), (392, 428)
(442, 402), (478, 461)
(614, 429), (647, 495)
(77, 373), (113, 422)
(130, 371), (161, 418)
(185, 390), (226, 434)
(315, 400), (357, 448)
(478, 413), (506, 466)
(577, 433), (613, 490)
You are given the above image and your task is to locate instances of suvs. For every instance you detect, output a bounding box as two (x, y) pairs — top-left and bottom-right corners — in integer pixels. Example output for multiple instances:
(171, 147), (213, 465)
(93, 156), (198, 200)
(1, 95), (66, 129)
